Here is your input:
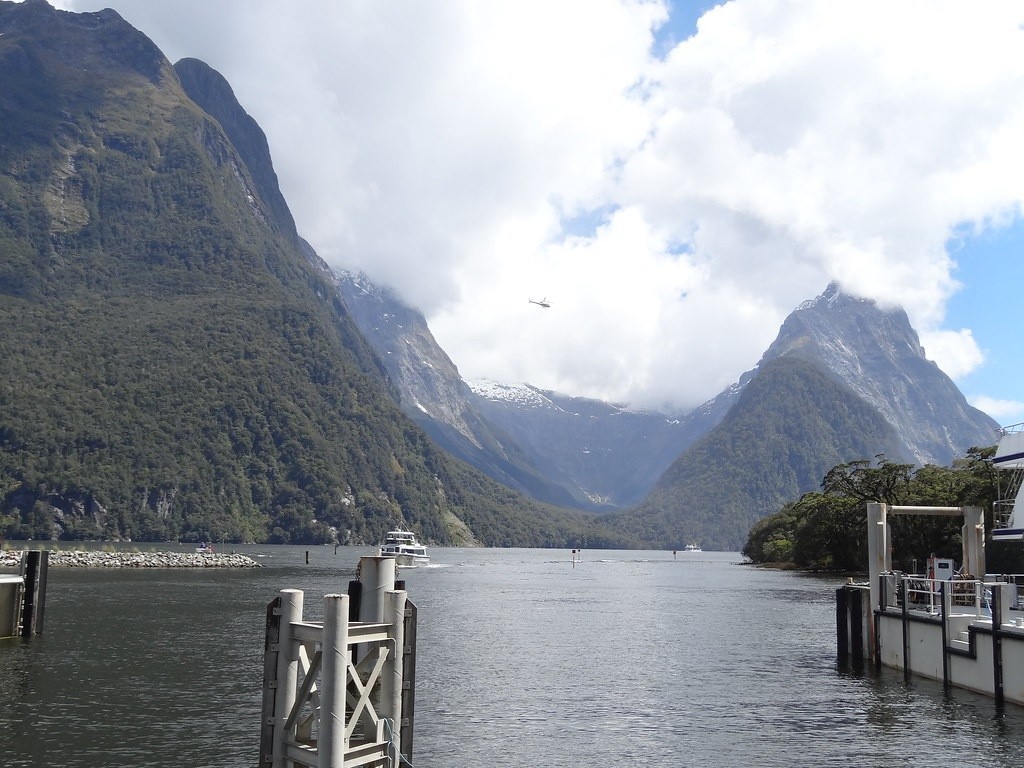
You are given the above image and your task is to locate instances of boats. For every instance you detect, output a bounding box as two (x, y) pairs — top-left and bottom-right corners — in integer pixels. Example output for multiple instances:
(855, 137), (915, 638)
(196, 546), (211, 553)
(684, 542), (701, 553)
(373, 529), (432, 567)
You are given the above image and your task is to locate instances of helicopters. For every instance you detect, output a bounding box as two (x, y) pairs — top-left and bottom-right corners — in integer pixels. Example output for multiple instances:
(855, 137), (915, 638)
(528, 296), (553, 311)
